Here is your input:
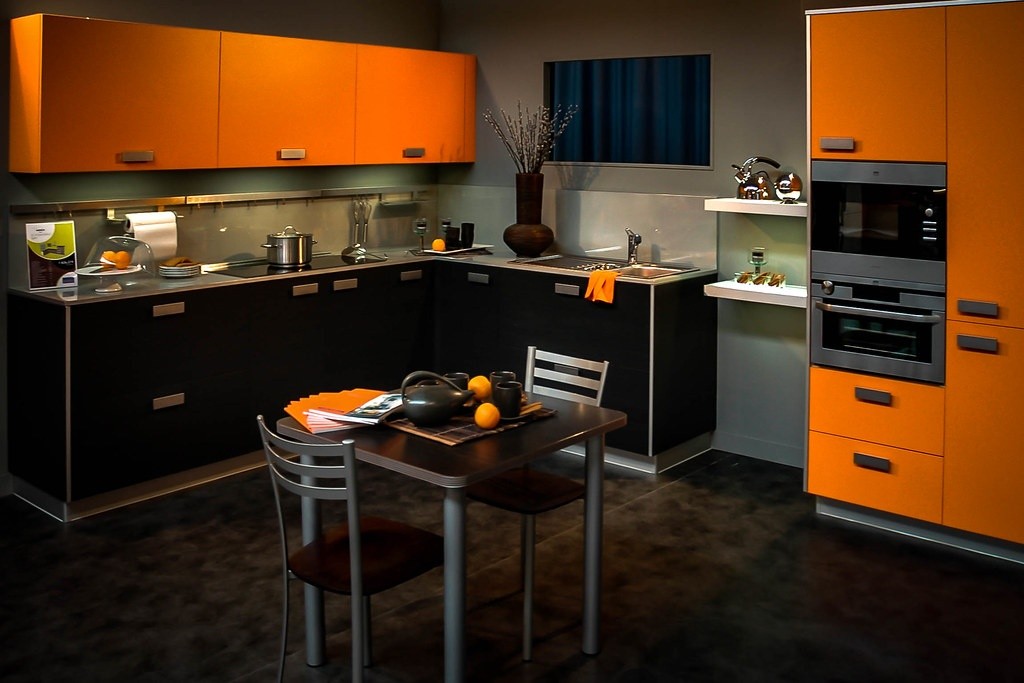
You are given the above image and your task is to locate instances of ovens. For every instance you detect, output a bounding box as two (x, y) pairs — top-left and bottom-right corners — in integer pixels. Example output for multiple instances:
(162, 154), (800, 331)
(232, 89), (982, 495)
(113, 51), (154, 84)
(811, 283), (945, 384)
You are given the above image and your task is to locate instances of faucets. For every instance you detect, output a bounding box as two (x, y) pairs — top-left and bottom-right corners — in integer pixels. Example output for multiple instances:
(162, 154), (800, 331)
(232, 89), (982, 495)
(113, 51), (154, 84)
(625, 227), (642, 263)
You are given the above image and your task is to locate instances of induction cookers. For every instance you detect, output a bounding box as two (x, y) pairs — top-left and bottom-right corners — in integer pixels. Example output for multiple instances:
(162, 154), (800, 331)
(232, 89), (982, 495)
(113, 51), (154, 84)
(203, 254), (386, 278)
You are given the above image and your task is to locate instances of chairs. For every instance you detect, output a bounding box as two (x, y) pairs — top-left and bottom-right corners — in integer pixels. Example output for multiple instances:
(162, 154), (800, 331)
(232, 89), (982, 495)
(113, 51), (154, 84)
(257, 415), (445, 683)
(466, 346), (609, 661)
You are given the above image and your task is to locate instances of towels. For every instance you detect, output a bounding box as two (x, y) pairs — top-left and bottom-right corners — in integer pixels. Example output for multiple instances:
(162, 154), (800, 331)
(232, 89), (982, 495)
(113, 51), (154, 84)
(584, 270), (623, 304)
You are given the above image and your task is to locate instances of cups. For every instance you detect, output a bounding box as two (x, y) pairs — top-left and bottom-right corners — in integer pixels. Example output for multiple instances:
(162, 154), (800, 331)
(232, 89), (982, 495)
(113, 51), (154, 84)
(442, 218), (451, 233)
(443, 372), (469, 391)
(490, 371), (516, 405)
(446, 226), (460, 250)
(460, 222), (475, 249)
(495, 380), (522, 418)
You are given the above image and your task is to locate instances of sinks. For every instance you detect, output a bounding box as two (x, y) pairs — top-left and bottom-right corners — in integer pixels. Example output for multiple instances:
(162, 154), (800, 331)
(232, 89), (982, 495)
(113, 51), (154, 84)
(612, 263), (701, 279)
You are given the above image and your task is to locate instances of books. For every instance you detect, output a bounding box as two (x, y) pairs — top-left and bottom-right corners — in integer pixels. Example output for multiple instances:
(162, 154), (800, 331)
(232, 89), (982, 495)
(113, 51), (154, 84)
(284, 388), (407, 436)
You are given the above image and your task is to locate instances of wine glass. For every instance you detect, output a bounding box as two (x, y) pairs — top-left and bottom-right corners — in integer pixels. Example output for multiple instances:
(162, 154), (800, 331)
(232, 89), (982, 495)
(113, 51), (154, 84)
(413, 217), (430, 254)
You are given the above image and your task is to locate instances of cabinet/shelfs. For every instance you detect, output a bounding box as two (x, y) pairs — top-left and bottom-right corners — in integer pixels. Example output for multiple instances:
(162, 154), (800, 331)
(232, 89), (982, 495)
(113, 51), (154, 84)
(704, 197), (809, 308)
(356, 43), (477, 164)
(803, 0), (1024, 567)
(217, 31), (355, 169)
(9, 13), (221, 173)
(7, 258), (718, 523)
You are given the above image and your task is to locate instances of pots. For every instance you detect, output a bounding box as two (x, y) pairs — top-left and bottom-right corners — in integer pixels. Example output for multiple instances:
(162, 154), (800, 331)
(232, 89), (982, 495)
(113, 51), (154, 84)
(261, 225), (317, 267)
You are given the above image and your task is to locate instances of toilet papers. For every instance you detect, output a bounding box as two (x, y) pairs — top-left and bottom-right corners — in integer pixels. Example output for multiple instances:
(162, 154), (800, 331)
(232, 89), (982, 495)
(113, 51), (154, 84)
(122, 210), (178, 264)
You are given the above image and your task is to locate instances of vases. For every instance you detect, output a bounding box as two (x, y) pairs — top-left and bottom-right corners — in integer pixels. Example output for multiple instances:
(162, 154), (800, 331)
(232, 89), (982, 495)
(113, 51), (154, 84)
(503, 171), (555, 258)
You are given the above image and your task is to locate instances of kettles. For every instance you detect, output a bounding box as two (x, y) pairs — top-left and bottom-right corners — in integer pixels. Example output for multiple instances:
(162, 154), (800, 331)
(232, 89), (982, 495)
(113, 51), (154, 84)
(731, 156), (781, 199)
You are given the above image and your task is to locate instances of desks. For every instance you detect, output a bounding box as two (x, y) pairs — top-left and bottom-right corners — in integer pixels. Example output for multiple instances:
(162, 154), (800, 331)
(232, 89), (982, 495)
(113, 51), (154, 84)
(276, 382), (628, 683)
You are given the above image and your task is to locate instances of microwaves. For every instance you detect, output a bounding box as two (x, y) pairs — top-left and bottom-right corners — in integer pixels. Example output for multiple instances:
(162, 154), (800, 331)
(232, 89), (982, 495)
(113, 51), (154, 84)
(811, 159), (947, 294)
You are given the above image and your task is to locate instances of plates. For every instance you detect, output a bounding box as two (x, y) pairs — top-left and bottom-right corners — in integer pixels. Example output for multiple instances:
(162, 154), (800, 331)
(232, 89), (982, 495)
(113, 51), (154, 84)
(159, 266), (198, 277)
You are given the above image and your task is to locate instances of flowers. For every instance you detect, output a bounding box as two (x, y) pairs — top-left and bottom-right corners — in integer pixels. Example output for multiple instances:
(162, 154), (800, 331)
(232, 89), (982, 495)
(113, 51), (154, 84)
(481, 99), (581, 174)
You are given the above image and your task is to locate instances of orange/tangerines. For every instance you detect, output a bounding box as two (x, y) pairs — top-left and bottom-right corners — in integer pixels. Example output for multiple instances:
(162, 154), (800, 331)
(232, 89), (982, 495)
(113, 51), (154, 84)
(468, 375), (491, 398)
(475, 403), (500, 428)
(432, 239), (445, 251)
(101, 250), (130, 268)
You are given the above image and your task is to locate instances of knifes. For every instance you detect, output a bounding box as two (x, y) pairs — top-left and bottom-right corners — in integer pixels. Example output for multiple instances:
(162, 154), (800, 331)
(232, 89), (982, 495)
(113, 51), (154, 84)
(89, 267), (114, 273)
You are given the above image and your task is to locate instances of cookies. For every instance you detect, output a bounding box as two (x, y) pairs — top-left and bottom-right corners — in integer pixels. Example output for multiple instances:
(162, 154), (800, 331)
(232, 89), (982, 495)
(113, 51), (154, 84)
(163, 257), (199, 267)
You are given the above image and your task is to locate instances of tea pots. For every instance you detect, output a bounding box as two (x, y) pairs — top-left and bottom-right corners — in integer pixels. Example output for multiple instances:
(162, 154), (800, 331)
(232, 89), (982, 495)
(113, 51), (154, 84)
(401, 371), (476, 427)
